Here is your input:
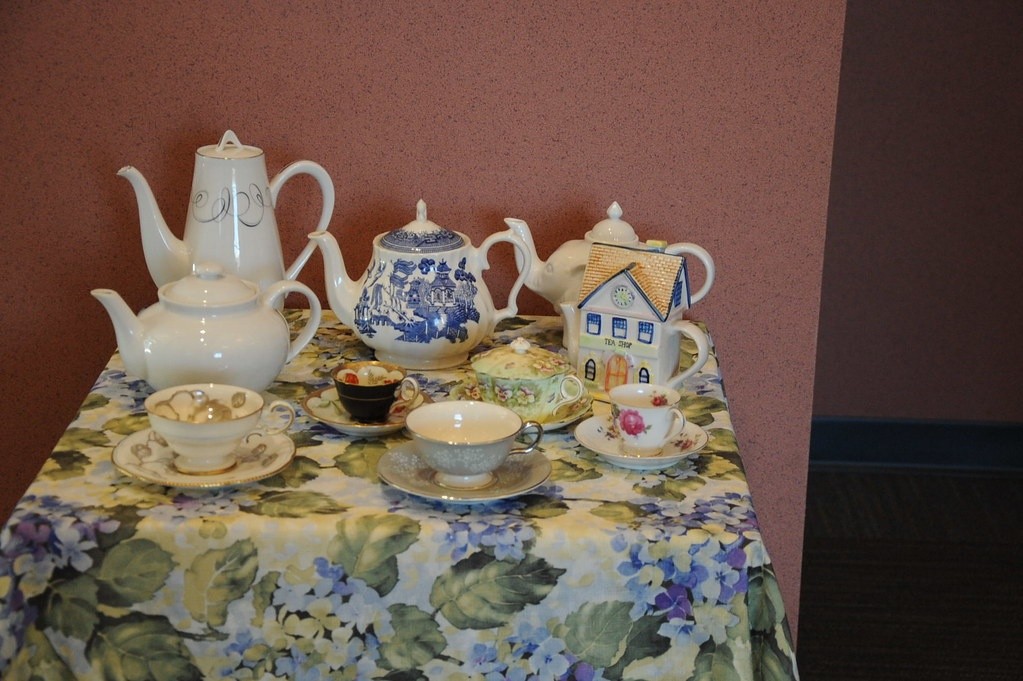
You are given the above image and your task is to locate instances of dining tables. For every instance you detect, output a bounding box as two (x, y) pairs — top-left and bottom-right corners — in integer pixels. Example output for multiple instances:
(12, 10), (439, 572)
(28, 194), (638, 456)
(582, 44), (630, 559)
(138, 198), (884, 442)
(1, 305), (803, 681)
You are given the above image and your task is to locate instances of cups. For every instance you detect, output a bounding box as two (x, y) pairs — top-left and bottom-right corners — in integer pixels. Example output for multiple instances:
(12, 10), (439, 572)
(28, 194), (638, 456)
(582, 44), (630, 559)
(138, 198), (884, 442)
(405, 400), (544, 490)
(607, 384), (685, 456)
(331, 361), (420, 424)
(471, 336), (582, 425)
(145, 383), (296, 478)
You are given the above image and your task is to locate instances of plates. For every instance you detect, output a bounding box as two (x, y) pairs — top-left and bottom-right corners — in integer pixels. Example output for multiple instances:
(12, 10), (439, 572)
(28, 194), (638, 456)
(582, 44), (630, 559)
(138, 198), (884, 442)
(574, 415), (710, 470)
(111, 429), (295, 491)
(451, 379), (594, 433)
(302, 384), (437, 437)
(377, 440), (552, 505)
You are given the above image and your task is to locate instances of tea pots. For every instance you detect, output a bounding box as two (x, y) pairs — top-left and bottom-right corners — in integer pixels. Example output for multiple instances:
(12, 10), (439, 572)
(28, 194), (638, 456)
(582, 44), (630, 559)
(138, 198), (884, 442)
(503, 200), (716, 349)
(560, 242), (709, 403)
(116, 130), (335, 312)
(90, 264), (321, 397)
(307, 198), (529, 370)
